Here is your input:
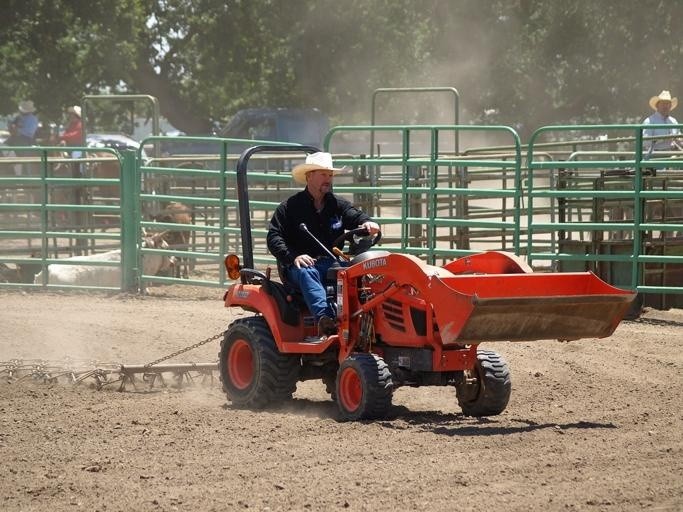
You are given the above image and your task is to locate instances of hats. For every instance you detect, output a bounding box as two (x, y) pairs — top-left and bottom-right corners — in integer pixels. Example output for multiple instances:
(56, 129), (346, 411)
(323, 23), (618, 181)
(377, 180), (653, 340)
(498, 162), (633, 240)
(18, 100), (37, 114)
(649, 90), (679, 112)
(291, 151), (350, 184)
(68, 106), (88, 121)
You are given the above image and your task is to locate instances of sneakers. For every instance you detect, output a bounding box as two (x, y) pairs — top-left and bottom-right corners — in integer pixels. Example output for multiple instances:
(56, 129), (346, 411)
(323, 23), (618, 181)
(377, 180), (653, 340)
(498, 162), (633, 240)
(316, 314), (338, 334)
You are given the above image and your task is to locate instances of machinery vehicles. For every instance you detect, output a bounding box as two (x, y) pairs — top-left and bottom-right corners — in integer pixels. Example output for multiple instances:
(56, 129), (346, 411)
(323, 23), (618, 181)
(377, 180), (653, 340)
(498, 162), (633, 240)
(0, 143), (639, 422)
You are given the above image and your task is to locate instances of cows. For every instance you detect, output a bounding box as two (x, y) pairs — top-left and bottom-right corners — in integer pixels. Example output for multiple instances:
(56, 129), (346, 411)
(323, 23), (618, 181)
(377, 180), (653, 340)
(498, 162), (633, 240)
(149, 202), (193, 279)
(33, 227), (178, 290)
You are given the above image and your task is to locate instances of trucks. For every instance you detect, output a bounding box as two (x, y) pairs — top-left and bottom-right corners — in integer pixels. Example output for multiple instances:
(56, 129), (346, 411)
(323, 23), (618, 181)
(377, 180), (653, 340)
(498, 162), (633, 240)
(148, 106), (413, 197)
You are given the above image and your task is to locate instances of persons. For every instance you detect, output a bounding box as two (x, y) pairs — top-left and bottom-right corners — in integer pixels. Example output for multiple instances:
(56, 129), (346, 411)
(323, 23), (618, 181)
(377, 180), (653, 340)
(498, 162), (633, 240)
(56, 104), (87, 176)
(638, 88), (682, 160)
(265, 151), (379, 336)
(0, 97), (38, 154)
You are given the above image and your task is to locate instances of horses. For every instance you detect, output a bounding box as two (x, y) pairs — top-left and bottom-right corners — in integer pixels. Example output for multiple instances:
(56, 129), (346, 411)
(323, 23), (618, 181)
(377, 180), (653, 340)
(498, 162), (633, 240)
(1, 125), (202, 233)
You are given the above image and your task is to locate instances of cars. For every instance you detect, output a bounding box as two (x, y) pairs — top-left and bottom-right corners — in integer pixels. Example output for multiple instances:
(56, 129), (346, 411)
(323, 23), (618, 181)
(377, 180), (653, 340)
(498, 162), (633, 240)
(0, 130), (149, 188)
